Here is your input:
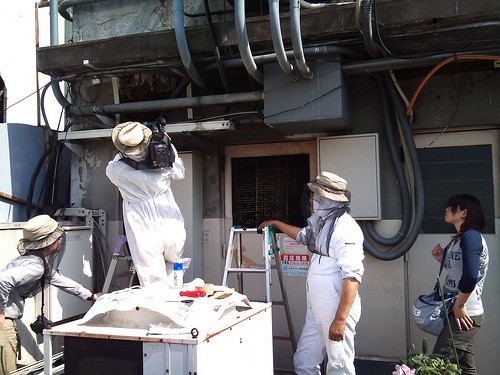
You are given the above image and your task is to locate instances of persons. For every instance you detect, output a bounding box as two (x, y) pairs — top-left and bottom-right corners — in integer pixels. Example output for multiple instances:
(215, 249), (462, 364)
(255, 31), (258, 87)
(258, 171), (364, 375)
(429, 194), (490, 375)
(0, 214), (104, 375)
(105, 122), (186, 288)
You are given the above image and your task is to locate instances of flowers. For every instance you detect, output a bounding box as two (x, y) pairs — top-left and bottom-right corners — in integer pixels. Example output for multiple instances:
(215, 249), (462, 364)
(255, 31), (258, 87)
(392, 337), (462, 375)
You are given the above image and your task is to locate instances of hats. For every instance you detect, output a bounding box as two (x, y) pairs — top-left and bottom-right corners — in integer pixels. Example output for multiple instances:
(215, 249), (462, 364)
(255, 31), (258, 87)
(308, 170), (350, 202)
(19, 214), (65, 250)
(112, 121), (153, 156)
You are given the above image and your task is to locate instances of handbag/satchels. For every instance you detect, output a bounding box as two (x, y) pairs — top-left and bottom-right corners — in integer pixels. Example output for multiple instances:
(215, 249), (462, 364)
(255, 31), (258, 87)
(411, 281), (459, 336)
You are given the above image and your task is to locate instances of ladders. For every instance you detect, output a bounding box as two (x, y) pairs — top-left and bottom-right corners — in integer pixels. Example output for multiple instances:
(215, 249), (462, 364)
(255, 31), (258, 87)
(220, 228), (297, 375)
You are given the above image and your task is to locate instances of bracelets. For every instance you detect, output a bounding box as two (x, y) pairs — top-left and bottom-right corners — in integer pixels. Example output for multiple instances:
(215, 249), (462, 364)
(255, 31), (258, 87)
(87, 291), (94, 301)
(455, 299), (467, 306)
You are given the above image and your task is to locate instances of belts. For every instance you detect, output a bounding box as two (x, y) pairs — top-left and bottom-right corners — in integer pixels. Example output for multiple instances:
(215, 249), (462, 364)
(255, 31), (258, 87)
(5, 315), (22, 320)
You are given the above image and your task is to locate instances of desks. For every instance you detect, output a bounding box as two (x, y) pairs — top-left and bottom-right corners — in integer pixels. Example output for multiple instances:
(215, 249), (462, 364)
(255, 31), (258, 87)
(42, 302), (274, 375)
